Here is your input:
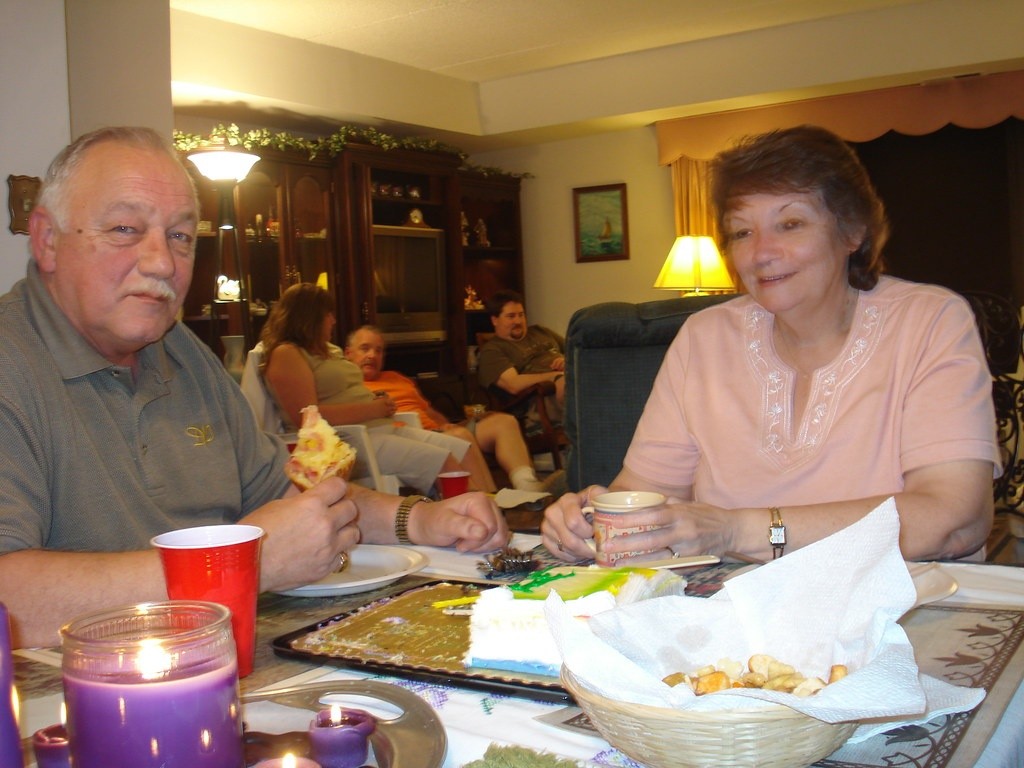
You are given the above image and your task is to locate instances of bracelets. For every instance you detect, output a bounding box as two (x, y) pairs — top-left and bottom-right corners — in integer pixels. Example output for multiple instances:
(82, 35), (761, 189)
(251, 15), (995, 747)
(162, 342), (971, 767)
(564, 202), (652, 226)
(339, 552), (348, 572)
(396, 495), (433, 545)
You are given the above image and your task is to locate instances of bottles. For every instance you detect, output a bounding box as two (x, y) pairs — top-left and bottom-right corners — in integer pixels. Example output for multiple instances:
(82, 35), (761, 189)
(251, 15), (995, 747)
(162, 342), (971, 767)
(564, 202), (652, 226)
(460, 211), (470, 245)
(474, 218), (487, 247)
(61, 599), (244, 768)
(362, 302), (371, 324)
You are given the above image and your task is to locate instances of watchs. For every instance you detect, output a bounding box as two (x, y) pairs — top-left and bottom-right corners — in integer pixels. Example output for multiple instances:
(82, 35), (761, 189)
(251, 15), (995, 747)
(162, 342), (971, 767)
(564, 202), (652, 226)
(770, 506), (786, 559)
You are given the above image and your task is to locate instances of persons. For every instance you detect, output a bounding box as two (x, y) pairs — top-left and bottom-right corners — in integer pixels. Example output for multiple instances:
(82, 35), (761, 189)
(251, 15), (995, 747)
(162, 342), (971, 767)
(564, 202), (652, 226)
(481, 289), (565, 421)
(540, 126), (1004, 561)
(0, 125), (511, 653)
(346, 325), (565, 510)
(257, 283), (489, 496)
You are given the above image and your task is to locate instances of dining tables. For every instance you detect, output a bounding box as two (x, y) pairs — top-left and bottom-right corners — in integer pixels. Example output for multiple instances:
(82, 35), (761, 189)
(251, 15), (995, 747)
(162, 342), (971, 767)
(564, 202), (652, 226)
(0, 532), (1024, 768)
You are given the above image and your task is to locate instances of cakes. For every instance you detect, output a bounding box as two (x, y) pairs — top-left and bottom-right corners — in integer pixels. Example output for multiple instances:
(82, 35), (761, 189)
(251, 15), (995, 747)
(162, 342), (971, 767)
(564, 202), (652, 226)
(461, 565), (688, 676)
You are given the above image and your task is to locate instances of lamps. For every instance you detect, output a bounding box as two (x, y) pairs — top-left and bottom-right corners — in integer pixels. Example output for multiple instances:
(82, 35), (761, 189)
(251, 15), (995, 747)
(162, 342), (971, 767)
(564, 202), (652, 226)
(186, 151), (262, 200)
(652, 234), (736, 297)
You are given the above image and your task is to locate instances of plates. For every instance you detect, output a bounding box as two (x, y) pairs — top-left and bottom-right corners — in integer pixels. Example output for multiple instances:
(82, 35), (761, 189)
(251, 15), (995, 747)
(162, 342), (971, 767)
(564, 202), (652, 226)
(276, 545), (431, 596)
(904, 560), (959, 610)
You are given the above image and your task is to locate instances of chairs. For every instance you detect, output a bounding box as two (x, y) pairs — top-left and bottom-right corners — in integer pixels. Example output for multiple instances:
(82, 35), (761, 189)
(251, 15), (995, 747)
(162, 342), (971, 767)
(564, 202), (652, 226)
(245, 347), (383, 492)
(475, 335), (567, 472)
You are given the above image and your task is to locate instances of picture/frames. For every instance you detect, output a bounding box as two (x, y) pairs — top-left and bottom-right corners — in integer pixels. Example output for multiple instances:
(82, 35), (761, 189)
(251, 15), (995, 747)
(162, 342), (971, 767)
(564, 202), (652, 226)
(572, 184), (631, 265)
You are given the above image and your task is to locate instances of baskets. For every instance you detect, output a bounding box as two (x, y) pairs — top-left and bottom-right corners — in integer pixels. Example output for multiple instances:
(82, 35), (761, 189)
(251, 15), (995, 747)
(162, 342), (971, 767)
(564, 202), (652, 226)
(560, 662), (859, 768)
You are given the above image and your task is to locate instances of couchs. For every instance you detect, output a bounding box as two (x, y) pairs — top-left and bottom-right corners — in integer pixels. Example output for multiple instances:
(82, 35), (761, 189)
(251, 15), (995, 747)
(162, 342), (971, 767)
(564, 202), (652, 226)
(563, 295), (738, 492)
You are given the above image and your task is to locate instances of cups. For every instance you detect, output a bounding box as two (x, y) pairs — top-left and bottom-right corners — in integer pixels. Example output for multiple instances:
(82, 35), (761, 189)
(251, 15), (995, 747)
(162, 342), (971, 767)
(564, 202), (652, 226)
(438, 471), (470, 499)
(220, 335), (244, 371)
(149, 522), (266, 676)
(581, 490), (666, 566)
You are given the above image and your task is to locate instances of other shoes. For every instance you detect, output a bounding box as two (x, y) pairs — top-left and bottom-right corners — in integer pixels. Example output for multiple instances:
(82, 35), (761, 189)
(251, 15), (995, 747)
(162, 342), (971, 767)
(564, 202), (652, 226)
(522, 469), (566, 512)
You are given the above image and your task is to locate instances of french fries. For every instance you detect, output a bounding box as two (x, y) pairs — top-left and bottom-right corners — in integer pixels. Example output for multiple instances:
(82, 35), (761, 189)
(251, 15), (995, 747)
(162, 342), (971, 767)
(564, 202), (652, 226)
(734, 665), (848, 696)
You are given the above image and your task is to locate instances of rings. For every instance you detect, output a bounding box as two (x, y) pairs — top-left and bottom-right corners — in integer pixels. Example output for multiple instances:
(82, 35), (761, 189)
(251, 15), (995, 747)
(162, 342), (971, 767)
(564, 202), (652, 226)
(558, 540), (562, 551)
(666, 547), (680, 559)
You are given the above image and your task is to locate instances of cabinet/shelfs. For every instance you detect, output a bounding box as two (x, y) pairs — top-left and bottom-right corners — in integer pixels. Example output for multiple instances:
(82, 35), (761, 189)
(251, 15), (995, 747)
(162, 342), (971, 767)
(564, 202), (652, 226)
(181, 141), (526, 377)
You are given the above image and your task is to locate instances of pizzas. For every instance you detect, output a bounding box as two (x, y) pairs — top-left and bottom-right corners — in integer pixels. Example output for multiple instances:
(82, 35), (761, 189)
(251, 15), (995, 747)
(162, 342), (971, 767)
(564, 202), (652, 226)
(284, 406), (358, 491)
